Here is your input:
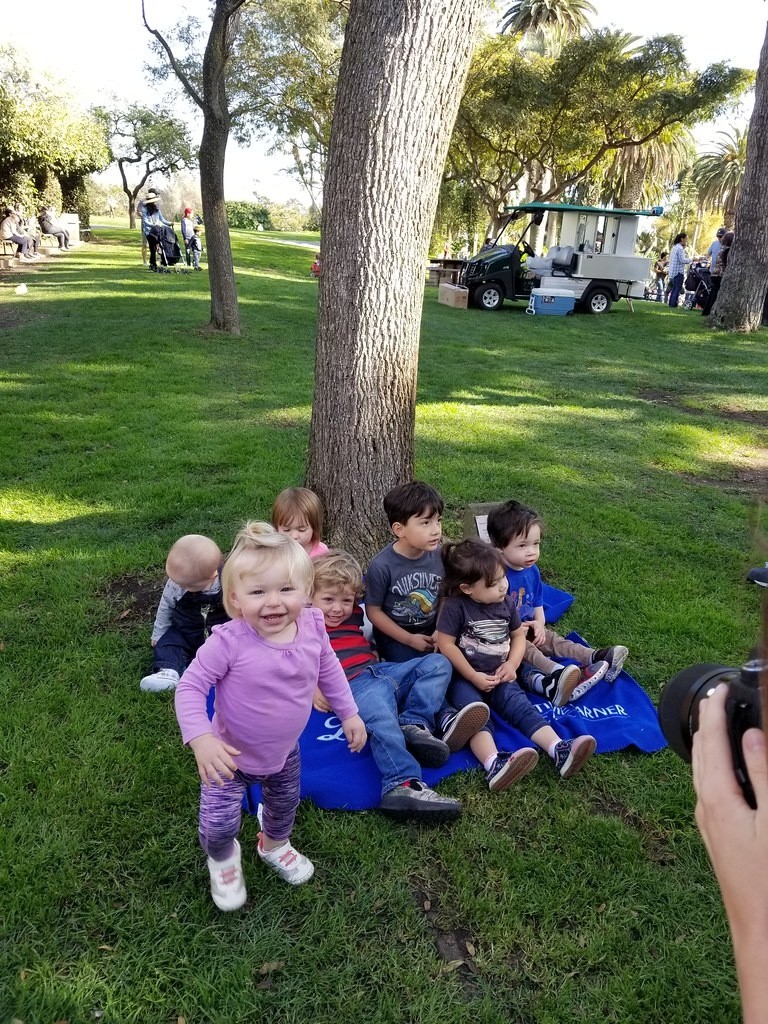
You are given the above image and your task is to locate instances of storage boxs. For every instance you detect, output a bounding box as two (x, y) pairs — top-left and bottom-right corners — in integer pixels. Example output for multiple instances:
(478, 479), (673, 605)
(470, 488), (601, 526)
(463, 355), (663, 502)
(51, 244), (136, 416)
(526, 257), (554, 270)
(439, 282), (471, 309)
(529, 287), (575, 316)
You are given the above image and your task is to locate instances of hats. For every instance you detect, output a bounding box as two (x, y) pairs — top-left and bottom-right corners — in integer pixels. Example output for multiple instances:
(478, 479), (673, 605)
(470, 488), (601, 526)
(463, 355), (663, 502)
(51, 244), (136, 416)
(184, 208), (192, 217)
(143, 192), (160, 205)
(717, 227), (726, 236)
(148, 188), (161, 195)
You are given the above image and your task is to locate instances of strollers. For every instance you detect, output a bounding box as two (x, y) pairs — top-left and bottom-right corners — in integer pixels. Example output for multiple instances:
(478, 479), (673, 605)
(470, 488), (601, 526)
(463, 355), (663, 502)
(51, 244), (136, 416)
(682, 261), (713, 310)
(153, 220), (190, 276)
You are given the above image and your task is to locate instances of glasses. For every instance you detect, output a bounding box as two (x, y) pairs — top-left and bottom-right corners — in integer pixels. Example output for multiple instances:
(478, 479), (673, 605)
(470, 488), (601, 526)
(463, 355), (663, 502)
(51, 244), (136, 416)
(717, 235), (724, 239)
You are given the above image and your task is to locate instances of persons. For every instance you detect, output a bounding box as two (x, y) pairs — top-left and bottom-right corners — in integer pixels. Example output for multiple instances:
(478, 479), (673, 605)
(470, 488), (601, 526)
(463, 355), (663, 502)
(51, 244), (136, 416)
(175, 519), (367, 912)
(108, 197), (116, 218)
(186, 227), (204, 272)
(691, 568), (768, 1023)
(669, 233), (699, 307)
(701, 228), (734, 316)
(362, 480), (581, 754)
(0, 203), (40, 260)
(437, 538), (597, 792)
(141, 193), (175, 271)
(36, 205), (70, 250)
(137, 188), (160, 266)
(140, 528), (231, 691)
(310, 548), (463, 822)
(272, 486), (328, 558)
(181, 208), (195, 266)
(487, 499), (628, 702)
(654, 252), (669, 303)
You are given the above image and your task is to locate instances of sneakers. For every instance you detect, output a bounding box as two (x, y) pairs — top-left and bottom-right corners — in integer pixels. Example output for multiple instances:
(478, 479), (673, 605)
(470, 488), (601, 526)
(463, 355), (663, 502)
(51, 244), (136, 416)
(397, 723), (452, 767)
(599, 645), (629, 683)
(541, 664), (581, 706)
(440, 701), (490, 753)
(553, 734), (597, 778)
(381, 779), (462, 820)
(256, 830), (315, 885)
(568, 661), (608, 701)
(484, 747), (538, 792)
(206, 838), (248, 911)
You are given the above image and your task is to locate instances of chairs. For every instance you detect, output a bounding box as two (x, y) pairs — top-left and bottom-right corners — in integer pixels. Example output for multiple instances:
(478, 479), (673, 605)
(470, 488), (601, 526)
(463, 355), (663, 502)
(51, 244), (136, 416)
(547, 245), (577, 277)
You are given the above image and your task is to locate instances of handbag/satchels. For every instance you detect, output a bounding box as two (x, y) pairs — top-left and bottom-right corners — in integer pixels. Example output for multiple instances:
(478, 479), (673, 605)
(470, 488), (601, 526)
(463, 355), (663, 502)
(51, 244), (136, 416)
(684, 269), (700, 291)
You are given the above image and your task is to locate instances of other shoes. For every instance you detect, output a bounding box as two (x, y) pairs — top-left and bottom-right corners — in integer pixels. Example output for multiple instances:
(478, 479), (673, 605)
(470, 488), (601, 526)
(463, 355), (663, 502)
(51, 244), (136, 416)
(149, 265), (157, 273)
(14, 245), (71, 263)
(193, 267), (202, 271)
(139, 668), (181, 693)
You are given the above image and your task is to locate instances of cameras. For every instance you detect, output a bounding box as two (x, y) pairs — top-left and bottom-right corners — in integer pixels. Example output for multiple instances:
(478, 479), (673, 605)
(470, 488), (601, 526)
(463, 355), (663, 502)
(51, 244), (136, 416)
(658, 562), (768, 808)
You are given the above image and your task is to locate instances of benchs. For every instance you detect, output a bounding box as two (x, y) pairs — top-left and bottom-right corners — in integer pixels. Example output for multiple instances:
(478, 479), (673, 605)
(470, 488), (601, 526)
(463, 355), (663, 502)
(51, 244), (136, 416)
(2, 234), (55, 255)
